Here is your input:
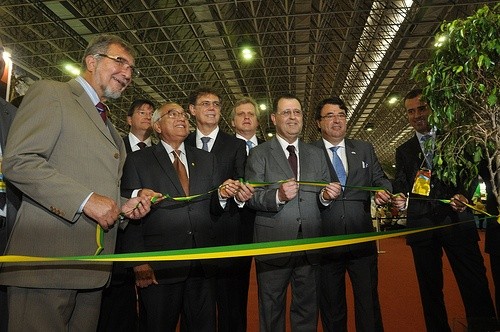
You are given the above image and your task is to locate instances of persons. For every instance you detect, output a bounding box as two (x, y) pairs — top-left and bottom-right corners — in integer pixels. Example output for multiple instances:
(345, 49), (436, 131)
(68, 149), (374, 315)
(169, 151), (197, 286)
(476, 198), (486, 232)
(120, 102), (239, 332)
(96, 100), (156, 332)
(231, 96), (266, 158)
(451, 139), (500, 321)
(246, 94), (341, 332)
(391, 91), (500, 332)
(185, 87), (254, 332)
(0, 34), (151, 332)
(309, 97), (393, 332)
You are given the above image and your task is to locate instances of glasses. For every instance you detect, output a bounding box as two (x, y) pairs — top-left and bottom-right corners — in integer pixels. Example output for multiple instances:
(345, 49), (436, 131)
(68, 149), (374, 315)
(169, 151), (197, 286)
(92, 53), (141, 78)
(153, 109), (192, 120)
(319, 114), (347, 120)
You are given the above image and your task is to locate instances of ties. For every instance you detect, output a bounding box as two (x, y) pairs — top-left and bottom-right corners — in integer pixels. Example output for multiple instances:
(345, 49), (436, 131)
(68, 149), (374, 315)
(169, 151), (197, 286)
(286, 145), (298, 181)
(171, 150), (190, 197)
(329, 145), (347, 191)
(137, 142), (146, 150)
(246, 141), (253, 156)
(420, 135), (433, 170)
(200, 137), (210, 152)
(97, 102), (107, 125)
